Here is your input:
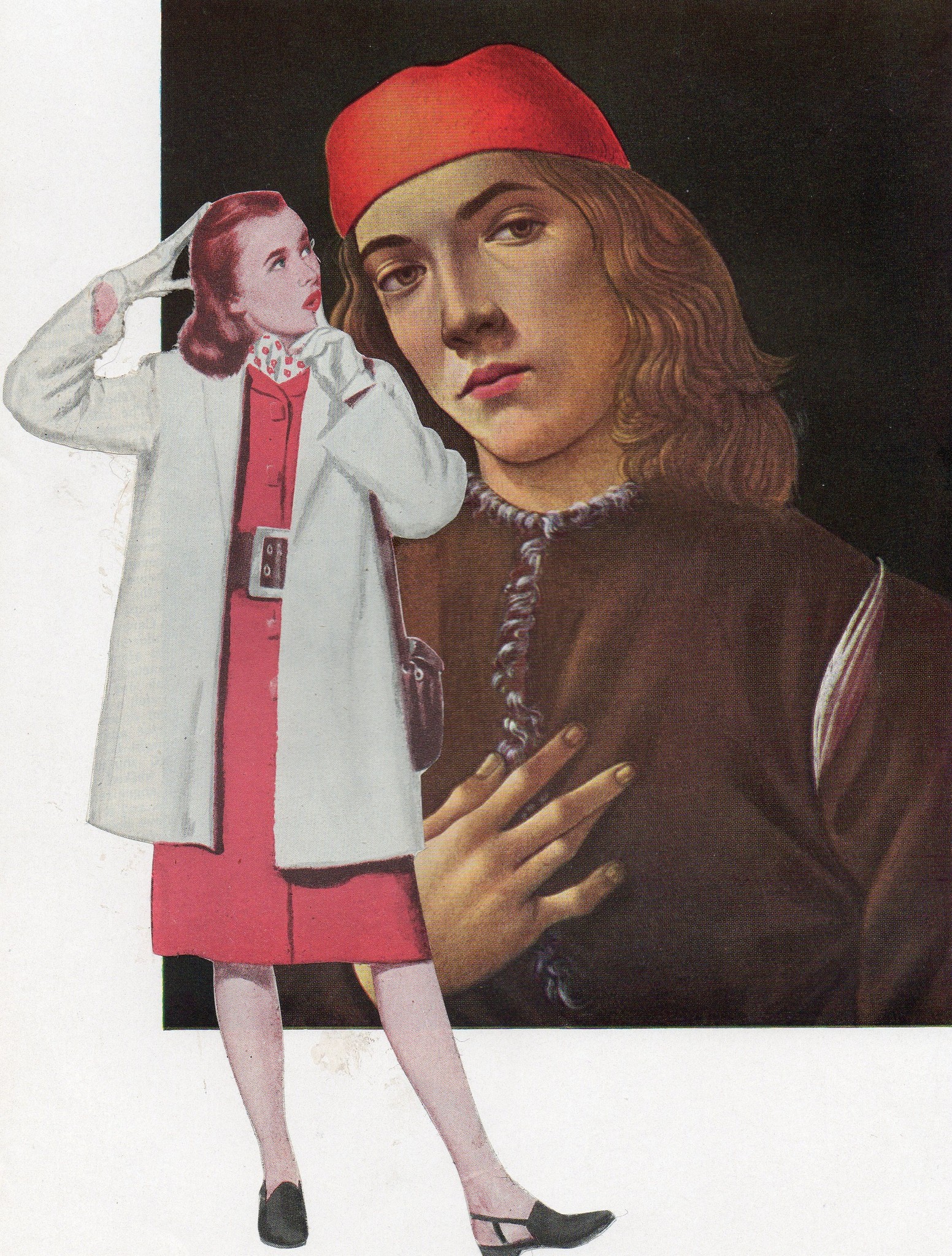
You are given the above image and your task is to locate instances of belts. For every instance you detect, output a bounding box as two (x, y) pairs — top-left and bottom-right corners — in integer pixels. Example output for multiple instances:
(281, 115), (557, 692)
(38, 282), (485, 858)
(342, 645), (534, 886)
(228, 526), (291, 598)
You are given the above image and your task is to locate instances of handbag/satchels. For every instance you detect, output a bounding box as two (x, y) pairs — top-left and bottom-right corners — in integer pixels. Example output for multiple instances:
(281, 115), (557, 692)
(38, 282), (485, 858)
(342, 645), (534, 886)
(397, 638), (446, 772)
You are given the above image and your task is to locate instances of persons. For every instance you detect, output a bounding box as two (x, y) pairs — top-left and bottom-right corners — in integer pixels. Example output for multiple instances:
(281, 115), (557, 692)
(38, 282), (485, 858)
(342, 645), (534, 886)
(0, 187), (616, 1256)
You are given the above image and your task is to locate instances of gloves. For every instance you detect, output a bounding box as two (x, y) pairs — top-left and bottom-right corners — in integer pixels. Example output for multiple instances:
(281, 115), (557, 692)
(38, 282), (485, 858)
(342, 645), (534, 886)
(101, 202), (212, 312)
(289, 327), (375, 403)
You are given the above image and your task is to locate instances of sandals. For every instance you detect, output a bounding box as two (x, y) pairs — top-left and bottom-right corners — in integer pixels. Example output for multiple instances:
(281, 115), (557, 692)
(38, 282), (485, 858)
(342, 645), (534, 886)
(471, 1200), (615, 1256)
(258, 1180), (308, 1248)
